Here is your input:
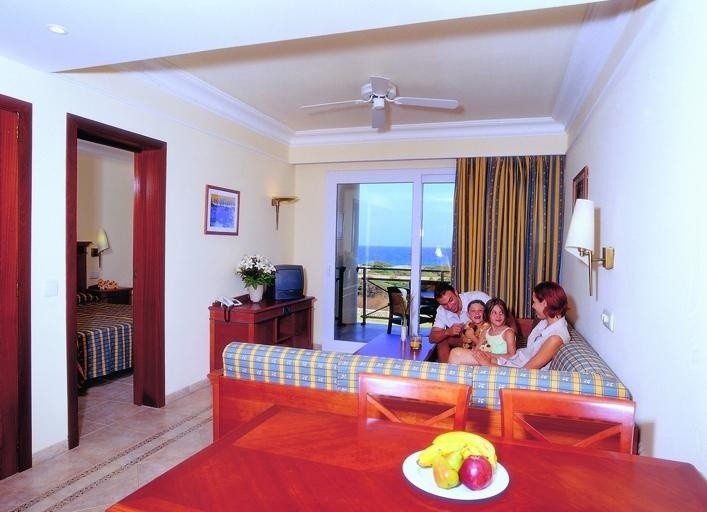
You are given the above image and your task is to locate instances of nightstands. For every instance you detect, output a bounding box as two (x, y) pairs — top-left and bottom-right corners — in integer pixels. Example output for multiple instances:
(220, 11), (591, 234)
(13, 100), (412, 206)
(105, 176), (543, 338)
(89, 285), (132, 304)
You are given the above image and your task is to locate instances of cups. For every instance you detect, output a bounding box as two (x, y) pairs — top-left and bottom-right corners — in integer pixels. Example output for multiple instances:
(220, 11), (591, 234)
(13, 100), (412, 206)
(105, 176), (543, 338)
(409, 335), (425, 351)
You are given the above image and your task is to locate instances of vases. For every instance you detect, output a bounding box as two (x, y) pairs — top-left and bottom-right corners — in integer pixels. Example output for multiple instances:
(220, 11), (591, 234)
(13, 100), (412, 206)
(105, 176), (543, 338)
(246, 284), (264, 303)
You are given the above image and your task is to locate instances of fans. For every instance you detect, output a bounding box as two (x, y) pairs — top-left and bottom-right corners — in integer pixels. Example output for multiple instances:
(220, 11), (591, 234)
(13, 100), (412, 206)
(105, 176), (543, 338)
(299, 74), (459, 129)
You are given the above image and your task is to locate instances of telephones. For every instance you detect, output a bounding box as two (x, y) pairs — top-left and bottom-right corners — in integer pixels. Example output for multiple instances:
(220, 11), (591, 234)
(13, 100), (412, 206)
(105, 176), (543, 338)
(215, 293), (243, 324)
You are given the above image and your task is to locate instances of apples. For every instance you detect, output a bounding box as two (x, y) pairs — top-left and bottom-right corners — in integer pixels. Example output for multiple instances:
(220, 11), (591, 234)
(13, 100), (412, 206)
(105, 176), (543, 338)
(459, 455), (492, 491)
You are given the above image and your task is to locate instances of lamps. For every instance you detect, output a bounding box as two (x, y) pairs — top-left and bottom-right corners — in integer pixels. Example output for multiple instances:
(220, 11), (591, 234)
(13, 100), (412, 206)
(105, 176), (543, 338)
(361, 82), (397, 111)
(270, 194), (297, 231)
(566, 200), (614, 296)
(94, 228), (109, 268)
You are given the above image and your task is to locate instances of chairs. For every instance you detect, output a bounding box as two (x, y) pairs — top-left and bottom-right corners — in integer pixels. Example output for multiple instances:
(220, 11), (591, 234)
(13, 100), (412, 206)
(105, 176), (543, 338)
(357, 371), (472, 432)
(409, 280), (449, 291)
(499, 387), (636, 454)
(386, 287), (434, 336)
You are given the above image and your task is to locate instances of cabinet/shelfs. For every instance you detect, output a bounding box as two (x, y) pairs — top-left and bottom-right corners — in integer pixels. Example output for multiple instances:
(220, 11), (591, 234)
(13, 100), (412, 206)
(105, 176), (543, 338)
(208, 294), (315, 372)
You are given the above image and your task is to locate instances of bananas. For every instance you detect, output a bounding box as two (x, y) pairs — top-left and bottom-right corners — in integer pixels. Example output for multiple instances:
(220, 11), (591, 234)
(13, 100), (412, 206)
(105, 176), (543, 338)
(417, 430), (498, 468)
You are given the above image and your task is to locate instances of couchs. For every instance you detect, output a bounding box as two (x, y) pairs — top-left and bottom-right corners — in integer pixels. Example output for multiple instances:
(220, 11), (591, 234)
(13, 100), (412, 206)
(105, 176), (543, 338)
(213, 319), (633, 444)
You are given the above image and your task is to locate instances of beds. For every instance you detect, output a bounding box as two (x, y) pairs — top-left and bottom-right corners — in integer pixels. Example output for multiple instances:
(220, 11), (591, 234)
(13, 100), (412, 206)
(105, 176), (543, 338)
(76, 301), (132, 397)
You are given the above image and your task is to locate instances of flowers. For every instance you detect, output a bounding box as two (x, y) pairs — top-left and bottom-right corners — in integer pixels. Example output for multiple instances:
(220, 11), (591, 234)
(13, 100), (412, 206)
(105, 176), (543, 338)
(233, 254), (277, 289)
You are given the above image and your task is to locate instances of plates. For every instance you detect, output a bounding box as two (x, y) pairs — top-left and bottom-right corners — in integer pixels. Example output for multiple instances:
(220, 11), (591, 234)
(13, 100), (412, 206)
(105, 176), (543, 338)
(403, 448), (510, 501)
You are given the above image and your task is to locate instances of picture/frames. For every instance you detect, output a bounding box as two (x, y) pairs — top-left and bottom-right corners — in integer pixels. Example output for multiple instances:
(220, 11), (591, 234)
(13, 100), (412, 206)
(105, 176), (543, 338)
(204, 184), (240, 236)
(572, 165), (590, 210)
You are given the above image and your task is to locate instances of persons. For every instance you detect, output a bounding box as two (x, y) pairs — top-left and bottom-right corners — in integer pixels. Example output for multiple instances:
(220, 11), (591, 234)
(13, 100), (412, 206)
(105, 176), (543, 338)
(467, 297), (517, 357)
(447, 281), (571, 370)
(459, 300), (491, 349)
(429, 283), (518, 367)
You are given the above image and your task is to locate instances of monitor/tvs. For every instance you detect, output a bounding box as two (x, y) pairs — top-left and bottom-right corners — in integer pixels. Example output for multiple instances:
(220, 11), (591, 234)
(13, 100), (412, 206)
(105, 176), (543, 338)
(272, 263), (304, 299)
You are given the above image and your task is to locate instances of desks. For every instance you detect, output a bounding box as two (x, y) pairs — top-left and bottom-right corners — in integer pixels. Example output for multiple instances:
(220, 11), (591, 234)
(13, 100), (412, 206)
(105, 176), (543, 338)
(420, 290), (435, 327)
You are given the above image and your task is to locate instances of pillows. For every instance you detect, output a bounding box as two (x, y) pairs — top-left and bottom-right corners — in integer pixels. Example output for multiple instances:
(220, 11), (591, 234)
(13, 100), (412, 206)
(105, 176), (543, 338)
(77, 292), (100, 303)
